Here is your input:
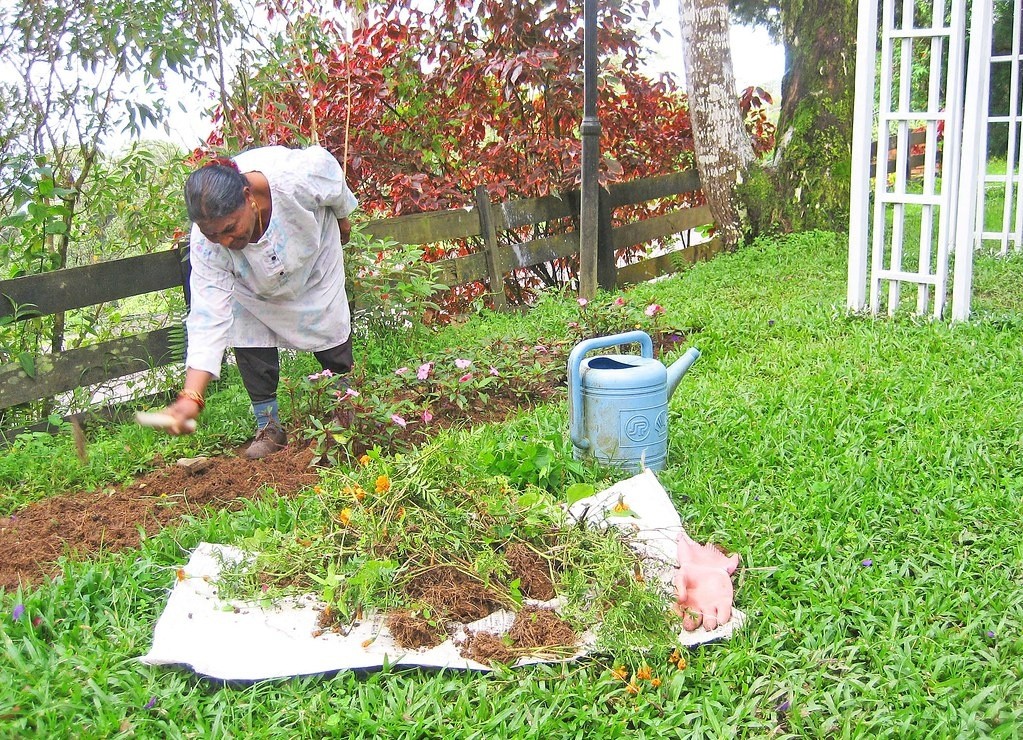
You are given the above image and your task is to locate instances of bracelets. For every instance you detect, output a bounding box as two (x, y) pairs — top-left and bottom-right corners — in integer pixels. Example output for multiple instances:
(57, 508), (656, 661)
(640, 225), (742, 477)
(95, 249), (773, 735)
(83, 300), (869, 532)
(179, 390), (205, 409)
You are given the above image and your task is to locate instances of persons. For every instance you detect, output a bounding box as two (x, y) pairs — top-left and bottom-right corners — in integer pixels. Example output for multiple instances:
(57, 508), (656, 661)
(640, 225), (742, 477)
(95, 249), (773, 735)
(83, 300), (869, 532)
(154, 146), (358, 458)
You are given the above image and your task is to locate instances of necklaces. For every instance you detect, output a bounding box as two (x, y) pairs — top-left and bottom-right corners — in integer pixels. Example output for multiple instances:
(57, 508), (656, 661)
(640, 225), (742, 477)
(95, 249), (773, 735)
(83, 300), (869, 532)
(254, 200), (263, 236)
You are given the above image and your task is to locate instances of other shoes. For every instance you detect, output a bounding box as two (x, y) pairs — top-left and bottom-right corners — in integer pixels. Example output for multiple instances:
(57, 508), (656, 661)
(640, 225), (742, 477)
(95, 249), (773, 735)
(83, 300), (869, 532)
(245, 427), (287, 459)
(332, 408), (355, 428)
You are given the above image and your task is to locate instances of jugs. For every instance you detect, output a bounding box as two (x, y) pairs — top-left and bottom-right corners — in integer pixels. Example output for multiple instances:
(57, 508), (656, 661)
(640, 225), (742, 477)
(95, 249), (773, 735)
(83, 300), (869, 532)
(567, 330), (700, 473)
